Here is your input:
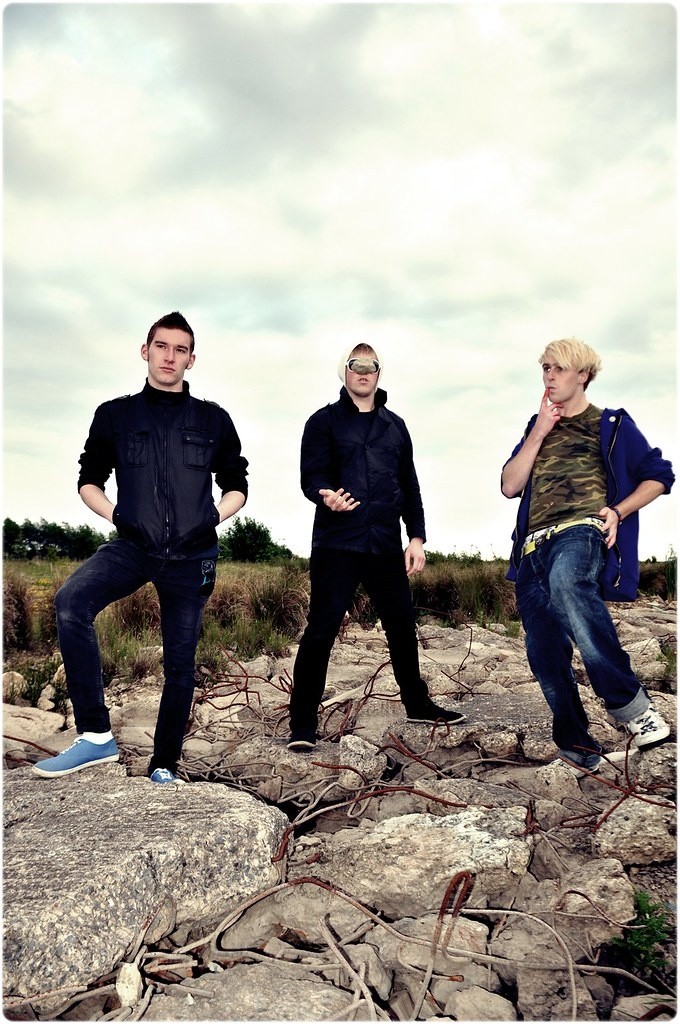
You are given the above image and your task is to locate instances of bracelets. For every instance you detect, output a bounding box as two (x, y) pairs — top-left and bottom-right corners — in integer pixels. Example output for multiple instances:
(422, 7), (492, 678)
(610, 507), (622, 526)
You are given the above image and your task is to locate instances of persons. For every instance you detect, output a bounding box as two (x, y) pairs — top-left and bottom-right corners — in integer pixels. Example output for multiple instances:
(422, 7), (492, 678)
(29, 312), (249, 784)
(284, 343), (466, 752)
(500, 338), (676, 770)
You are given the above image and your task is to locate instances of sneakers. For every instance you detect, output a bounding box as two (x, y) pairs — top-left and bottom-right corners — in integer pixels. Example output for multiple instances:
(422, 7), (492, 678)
(150, 768), (188, 785)
(31, 737), (120, 778)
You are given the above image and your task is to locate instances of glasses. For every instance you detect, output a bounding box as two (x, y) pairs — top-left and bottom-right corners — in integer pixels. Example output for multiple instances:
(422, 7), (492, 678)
(345, 357), (380, 375)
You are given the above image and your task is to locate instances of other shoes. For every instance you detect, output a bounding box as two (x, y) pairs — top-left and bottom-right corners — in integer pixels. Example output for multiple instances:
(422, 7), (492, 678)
(628, 701), (671, 752)
(403, 705), (465, 726)
(286, 728), (317, 748)
(534, 758), (600, 779)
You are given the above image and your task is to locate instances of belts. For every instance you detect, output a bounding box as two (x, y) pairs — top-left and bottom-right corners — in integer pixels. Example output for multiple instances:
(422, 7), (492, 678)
(520, 517), (607, 557)
